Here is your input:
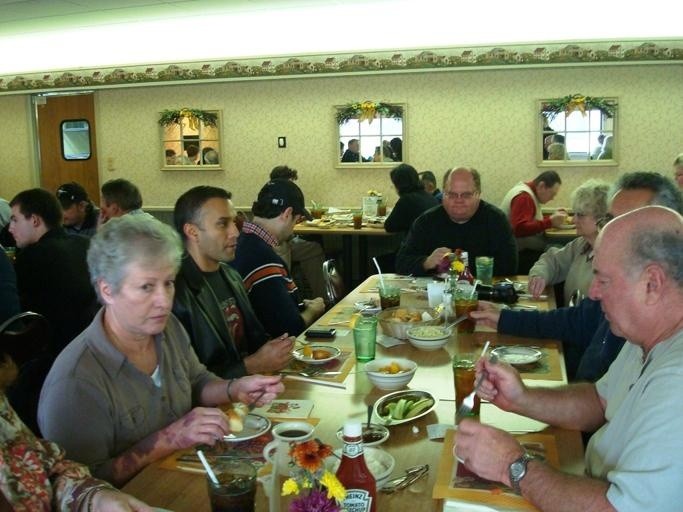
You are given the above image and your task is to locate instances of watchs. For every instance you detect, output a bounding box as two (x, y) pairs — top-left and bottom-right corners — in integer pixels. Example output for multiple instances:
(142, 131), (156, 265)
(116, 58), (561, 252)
(509, 452), (536, 495)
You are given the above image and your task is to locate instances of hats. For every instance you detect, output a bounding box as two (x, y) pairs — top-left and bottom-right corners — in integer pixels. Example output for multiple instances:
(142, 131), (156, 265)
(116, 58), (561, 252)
(258, 179), (312, 221)
(57, 182), (86, 200)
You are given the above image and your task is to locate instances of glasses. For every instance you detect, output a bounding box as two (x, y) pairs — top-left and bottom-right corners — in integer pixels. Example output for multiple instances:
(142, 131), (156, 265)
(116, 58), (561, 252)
(380, 465), (429, 495)
(445, 191), (475, 199)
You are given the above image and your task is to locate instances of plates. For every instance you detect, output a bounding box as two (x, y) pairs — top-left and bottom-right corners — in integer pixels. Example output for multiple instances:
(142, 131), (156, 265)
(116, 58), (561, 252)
(354, 303), (382, 315)
(491, 345), (541, 364)
(337, 422), (389, 445)
(216, 412), (271, 442)
(373, 390), (440, 425)
(556, 225), (576, 229)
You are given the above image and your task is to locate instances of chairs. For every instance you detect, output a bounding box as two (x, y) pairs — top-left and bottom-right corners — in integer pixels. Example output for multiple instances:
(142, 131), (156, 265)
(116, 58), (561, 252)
(322, 259), (350, 306)
(369, 253), (398, 275)
(1, 312), (47, 371)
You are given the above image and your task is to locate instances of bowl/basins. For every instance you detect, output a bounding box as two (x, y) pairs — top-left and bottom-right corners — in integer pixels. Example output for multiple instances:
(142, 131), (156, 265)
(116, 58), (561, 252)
(292, 346), (341, 364)
(365, 358), (417, 390)
(406, 326), (453, 351)
(325, 448), (395, 492)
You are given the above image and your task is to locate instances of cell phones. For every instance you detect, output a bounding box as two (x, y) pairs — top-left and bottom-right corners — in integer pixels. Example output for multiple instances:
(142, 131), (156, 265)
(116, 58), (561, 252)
(304, 328), (337, 339)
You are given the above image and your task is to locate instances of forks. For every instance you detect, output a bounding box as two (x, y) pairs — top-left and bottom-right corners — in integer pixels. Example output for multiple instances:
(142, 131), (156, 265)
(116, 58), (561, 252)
(458, 348), (504, 417)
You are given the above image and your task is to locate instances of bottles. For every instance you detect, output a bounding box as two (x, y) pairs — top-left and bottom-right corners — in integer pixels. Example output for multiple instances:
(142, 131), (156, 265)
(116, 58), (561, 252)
(458, 251), (473, 285)
(336, 423), (377, 512)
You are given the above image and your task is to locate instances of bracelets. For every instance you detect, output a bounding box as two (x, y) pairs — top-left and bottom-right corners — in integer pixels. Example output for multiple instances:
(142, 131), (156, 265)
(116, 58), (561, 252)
(225, 377), (236, 406)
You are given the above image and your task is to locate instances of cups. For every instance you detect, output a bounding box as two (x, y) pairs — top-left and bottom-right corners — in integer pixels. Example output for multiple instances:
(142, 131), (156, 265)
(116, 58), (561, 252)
(454, 352), (482, 416)
(353, 317), (377, 361)
(379, 285), (401, 310)
(311, 196), (386, 230)
(476, 256), (494, 285)
(455, 291), (478, 333)
(206, 460), (257, 512)
(427, 283), (445, 308)
(263, 420), (315, 476)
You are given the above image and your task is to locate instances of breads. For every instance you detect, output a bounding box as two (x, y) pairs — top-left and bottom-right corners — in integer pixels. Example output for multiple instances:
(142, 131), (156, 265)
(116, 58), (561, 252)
(216, 404), (249, 432)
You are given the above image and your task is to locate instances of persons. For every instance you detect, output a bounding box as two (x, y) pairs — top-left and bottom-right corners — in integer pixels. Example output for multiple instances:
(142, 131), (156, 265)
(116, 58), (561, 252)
(170, 184), (297, 382)
(229, 177), (327, 340)
(166, 144), (217, 166)
(393, 166), (520, 276)
(672, 153), (683, 191)
(37, 213), (285, 491)
(96, 178), (143, 233)
(454, 205), (681, 511)
(339, 137), (443, 242)
(1, 355), (171, 512)
(543, 115), (570, 160)
(592, 134), (613, 159)
(269, 167), (332, 312)
(501, 171), (568, 254)
(530, 181), (611, 308)
(56, 181), (108, 242)
(470, 171), (681, 452)
(0, 187), (103, 438)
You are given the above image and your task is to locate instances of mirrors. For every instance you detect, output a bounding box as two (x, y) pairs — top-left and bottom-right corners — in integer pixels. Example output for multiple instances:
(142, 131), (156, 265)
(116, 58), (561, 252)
(60, 118), (92, 161)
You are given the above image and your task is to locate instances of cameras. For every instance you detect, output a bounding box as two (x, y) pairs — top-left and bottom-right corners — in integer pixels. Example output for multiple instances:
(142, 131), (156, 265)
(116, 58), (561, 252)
(476, 282), (522, 304)
